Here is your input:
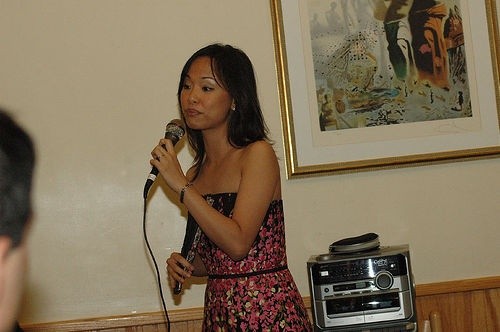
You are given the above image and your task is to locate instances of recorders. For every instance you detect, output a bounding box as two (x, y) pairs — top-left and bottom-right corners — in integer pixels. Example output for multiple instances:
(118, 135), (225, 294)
(307, 244), (415, 330)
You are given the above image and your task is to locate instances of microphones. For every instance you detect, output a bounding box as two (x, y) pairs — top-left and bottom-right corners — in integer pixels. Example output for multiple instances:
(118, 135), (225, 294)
(143, 119), (186, 198)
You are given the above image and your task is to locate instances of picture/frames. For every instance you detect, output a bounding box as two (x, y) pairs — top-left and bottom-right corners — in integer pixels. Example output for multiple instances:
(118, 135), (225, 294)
(268, 0), (500, 181)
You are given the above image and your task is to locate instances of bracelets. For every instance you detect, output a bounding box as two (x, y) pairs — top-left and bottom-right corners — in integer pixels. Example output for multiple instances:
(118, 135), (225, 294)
(178, 181), (193, 204)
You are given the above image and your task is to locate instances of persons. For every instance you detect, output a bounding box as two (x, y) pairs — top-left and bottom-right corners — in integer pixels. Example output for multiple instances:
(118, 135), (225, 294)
(0, 108), (36, 332)
(150, 43), (314, 332)
